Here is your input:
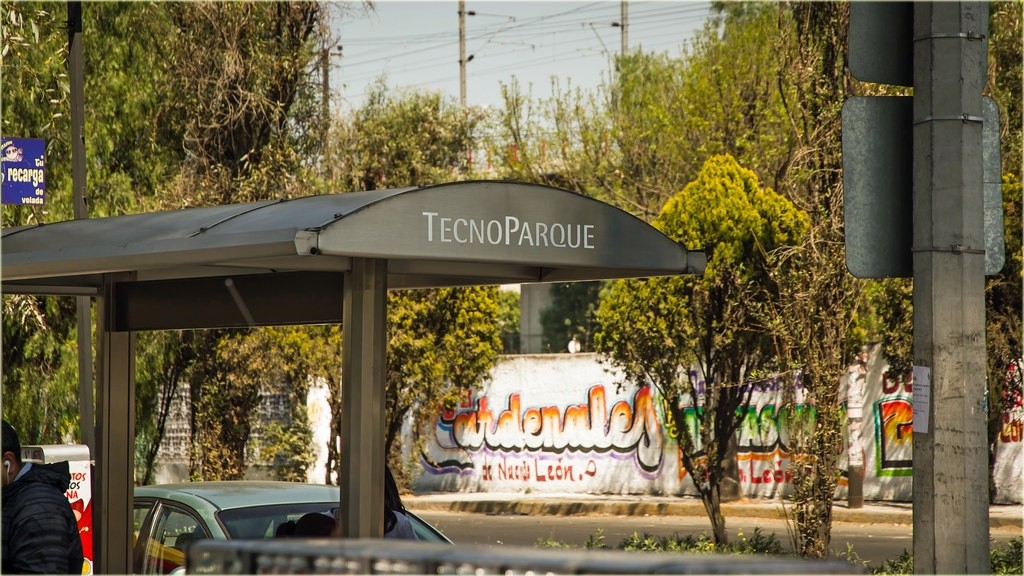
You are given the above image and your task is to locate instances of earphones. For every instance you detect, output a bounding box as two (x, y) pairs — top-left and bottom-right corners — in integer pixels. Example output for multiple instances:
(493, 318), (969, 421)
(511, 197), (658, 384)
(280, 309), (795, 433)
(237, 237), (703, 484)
(4, 460), (11, 473)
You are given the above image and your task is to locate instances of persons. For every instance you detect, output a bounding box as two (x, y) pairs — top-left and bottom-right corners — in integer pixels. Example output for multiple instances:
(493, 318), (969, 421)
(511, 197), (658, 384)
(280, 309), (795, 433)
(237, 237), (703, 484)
(276, 462), (424, 543)
(0, 419), (83, 575)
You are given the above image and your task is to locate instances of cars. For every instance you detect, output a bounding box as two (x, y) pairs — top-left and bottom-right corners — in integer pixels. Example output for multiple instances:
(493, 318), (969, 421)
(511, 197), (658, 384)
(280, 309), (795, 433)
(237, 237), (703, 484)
(126, 479), (463, 575)
